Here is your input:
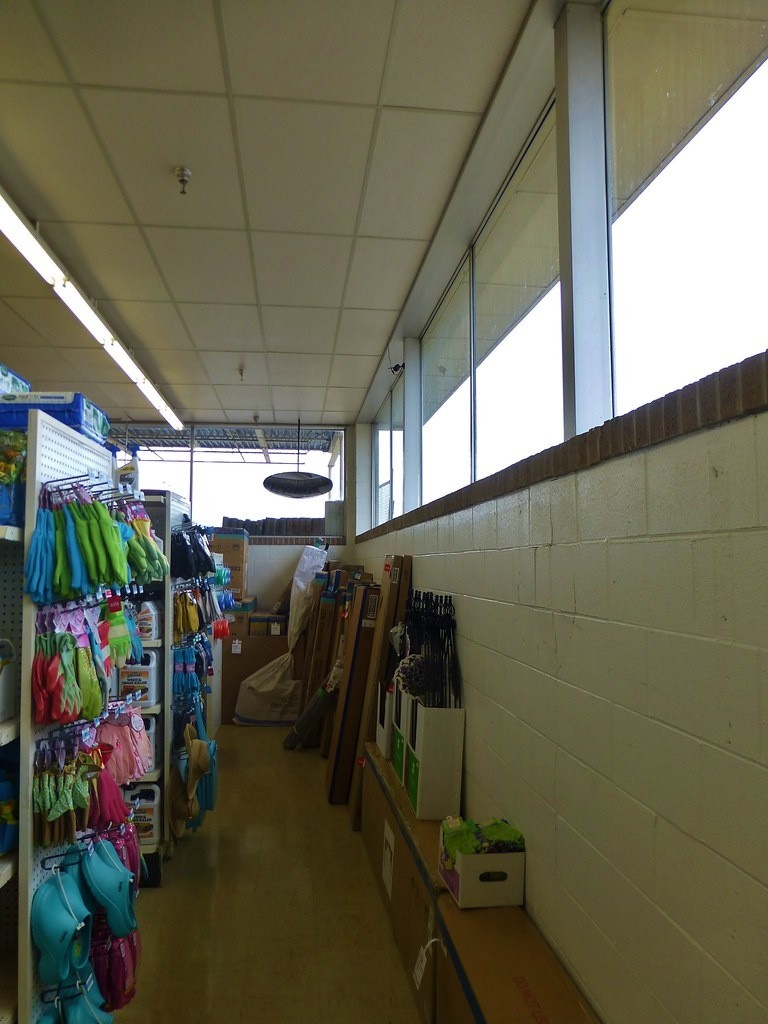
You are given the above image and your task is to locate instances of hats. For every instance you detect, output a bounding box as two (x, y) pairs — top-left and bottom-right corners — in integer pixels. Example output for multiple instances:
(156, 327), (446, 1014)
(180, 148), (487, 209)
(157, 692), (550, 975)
(183, 723), (211, 799)
(170, 766), (200, 837)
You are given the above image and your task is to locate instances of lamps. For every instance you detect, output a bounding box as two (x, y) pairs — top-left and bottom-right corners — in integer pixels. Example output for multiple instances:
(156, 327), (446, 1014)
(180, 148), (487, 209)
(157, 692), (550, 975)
(0, 183), (185, 432)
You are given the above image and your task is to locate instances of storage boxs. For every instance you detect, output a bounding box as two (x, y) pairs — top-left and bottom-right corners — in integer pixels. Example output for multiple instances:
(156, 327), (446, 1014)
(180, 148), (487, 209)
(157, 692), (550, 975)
(207, 527), (602, 1024)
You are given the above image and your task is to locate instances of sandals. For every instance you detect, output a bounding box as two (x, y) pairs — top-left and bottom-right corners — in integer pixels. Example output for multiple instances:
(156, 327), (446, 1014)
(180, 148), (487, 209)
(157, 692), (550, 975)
(30, 872), (115, 1024)
(82, 839), (141, 937)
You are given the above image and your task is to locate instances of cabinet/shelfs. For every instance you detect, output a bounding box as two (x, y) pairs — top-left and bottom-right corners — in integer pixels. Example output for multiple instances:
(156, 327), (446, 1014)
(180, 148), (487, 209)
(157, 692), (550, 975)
(104, 493), (165, 854)
(0, 525), (23, 1024)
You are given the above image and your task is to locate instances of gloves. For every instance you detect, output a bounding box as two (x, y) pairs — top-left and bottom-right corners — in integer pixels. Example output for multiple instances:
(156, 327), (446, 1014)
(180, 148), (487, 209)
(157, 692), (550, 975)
(20, 498), (170, 851)
